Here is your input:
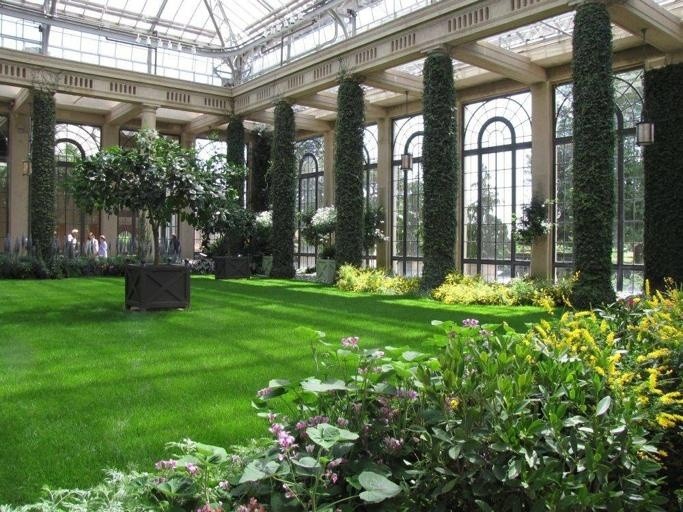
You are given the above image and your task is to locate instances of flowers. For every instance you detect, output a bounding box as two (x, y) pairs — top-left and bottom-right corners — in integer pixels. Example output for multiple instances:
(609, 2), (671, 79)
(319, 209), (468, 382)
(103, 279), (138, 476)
(252, 203), (273, 227)
(55, 120), (251, 264)
(311, 204), (337, 259)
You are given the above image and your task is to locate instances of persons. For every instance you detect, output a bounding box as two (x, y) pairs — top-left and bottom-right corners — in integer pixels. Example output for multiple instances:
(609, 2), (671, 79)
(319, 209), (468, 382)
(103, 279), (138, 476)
(3, 229), (109, 261)
(116, 232), (182, 257)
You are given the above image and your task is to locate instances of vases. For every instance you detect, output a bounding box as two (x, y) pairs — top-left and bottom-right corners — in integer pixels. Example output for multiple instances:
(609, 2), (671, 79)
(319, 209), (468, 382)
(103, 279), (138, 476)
(125, 259), (190, 312)
(262, 255), (273, 276)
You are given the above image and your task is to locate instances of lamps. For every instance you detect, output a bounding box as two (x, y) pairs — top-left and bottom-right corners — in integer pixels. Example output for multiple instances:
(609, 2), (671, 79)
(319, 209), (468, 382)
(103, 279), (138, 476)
(136, 34), (197, 55)
(636, 28), (656, 146)
(21, 152), (33, 175)
(400, 153), (413, 170)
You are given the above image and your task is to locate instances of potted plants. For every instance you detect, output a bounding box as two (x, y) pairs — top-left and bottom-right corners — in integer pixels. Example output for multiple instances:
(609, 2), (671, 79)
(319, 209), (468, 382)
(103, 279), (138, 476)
(315, 241), (339, 285)
(208, 234), (252, 279)
